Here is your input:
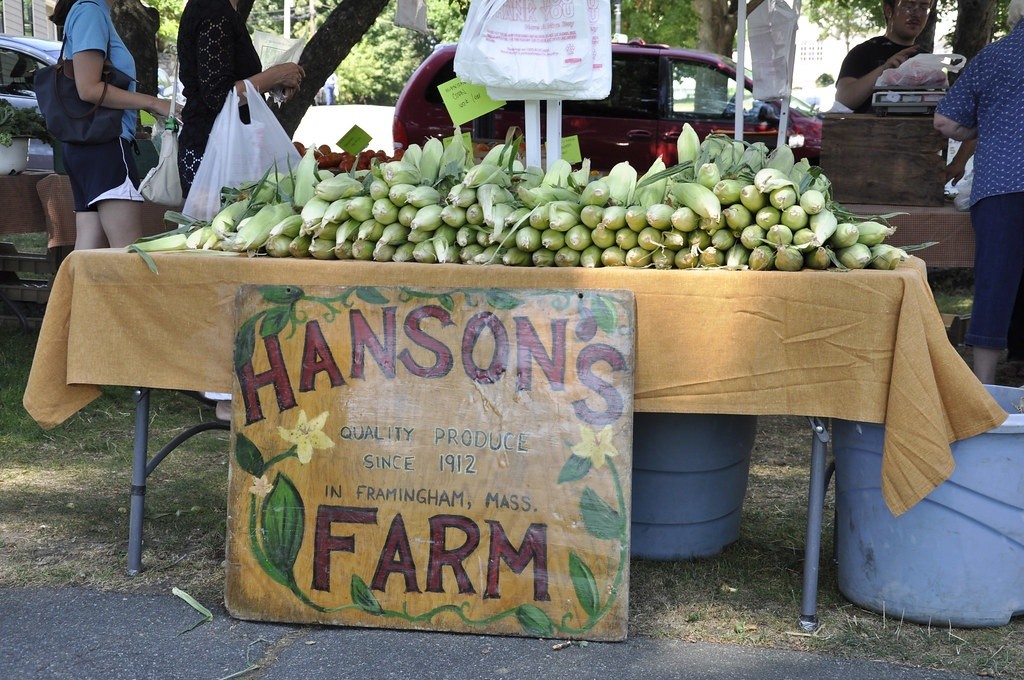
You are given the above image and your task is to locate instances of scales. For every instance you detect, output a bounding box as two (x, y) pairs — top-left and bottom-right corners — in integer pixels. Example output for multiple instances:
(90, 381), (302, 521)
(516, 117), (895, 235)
(872, 85), (948, 115)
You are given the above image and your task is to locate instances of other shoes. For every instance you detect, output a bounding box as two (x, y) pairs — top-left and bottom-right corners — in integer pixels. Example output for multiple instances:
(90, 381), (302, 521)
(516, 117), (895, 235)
(215, 398), (233, 420)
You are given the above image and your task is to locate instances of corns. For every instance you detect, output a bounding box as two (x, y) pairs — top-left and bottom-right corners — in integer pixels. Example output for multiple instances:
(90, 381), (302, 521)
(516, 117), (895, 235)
(127, 122), (900, 271)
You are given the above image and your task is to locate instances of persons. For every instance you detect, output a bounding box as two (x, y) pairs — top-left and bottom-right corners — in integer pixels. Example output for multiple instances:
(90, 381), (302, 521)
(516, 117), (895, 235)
(177, 0), (305, 199)
(835, 0), (933, 114)
(48, 0), (183, 250)
(934, 0), (1024, 385)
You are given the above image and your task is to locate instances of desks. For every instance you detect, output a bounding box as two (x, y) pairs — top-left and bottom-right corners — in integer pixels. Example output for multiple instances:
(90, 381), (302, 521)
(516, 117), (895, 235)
(839, 204), (976, 268)
(24, 243), (1012, 632)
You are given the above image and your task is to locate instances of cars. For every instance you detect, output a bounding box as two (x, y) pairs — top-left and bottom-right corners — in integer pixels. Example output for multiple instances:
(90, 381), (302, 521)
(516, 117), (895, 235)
(393, 41), (822, 182)
(0, 34), (62, 118)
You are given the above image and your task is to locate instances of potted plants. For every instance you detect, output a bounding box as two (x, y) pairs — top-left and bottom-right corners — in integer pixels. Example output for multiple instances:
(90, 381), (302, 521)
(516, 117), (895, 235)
(0, 98), (54, 176)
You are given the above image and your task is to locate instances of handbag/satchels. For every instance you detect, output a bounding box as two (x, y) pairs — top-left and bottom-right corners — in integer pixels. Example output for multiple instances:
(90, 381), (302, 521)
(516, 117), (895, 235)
(179, 79), (303, 232)
(875, 54), (966, 89)
(32, 2), (139, 144)
(142, 128), (186, 207)
